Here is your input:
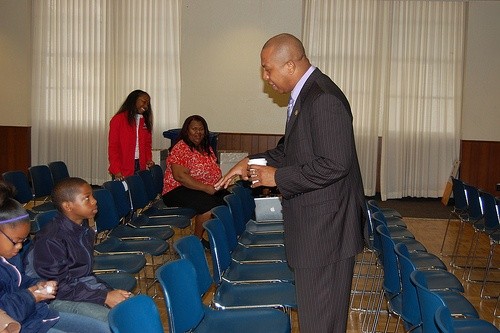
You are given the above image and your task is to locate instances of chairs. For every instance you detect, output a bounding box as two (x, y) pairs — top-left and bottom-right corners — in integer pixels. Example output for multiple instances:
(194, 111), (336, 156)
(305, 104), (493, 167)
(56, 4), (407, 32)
(0, 161), (500, 333)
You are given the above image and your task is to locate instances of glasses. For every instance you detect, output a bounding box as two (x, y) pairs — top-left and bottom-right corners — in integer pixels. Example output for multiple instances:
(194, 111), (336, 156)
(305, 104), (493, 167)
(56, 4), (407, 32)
(0, 229), (30, 246)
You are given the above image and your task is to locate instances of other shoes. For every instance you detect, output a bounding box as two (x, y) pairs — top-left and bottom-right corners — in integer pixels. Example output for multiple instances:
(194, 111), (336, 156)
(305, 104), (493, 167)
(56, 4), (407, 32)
(200, 238), (210, 252)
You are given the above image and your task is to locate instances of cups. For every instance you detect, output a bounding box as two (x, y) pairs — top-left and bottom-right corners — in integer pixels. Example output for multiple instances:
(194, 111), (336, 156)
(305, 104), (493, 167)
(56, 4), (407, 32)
(247, 157), (267, 185)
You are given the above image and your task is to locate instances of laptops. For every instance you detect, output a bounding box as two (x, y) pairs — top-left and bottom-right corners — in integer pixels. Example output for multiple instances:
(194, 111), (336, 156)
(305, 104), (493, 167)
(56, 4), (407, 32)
(253, 196), (284, 221)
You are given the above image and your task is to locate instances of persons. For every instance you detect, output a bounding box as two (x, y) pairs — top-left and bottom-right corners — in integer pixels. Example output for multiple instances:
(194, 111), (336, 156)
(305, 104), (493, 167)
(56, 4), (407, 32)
(108, 90), (155, 181)
(163, 115), (232, 249)
(24, 177), (132, 323)
(1, 178), (111, 333)
(214, 33), (370, 333)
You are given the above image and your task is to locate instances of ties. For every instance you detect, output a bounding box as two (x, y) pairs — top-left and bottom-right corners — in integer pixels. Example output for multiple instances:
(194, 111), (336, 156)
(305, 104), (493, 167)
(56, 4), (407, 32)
(287, 96), (295, 122)
